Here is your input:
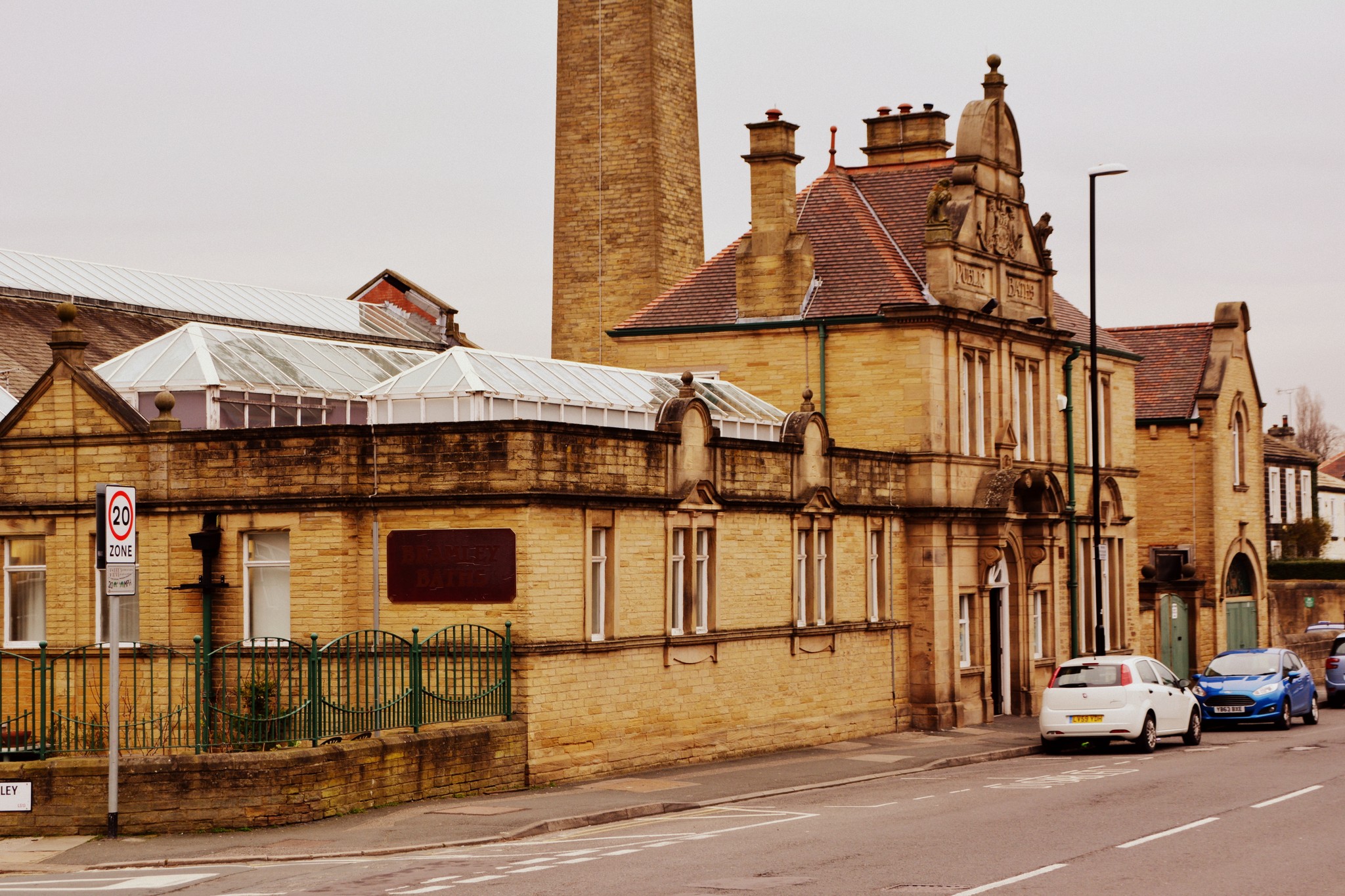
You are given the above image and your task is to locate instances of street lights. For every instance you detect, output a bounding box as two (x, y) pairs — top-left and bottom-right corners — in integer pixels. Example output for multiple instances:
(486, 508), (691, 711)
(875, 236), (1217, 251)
(1085, 162), (1129, 652)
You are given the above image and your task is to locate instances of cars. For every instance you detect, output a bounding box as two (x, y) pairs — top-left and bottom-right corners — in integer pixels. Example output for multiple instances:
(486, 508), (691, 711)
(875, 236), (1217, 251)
(1036, 654), (1203, 754)
(1190, 647), (1320, 729)
(1323, 632), (1345, 707)
(1303, 620), (1345, 633)
